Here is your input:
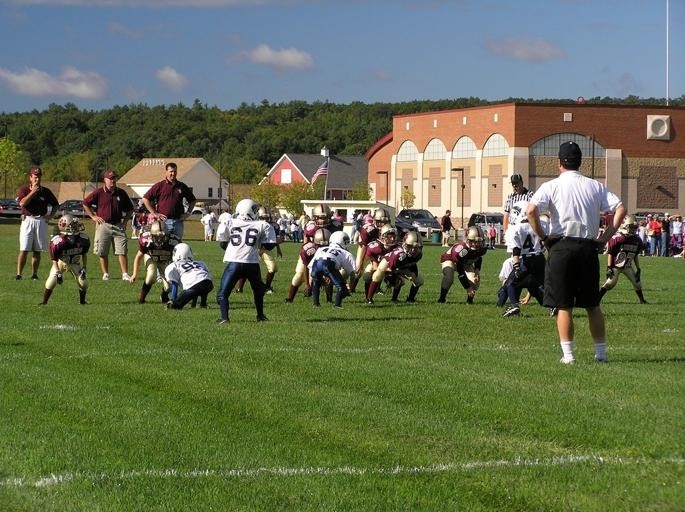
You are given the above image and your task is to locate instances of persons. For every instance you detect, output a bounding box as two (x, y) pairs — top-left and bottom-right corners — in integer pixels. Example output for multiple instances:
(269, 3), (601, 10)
(130, 220), (170, 305)
(215, 197), (277, 327)
(128, 199), (158, 240)
(596, 211), (685, 303)
(141, 161), (198, 283)
(501, 172), (533, 239)
(198, 205), (424, 309)
(438, 210), (532, 310)
(14, 166), (59, 279)
(36, 213), (90, 306)
(126, 212), (158, 284)
(82, 170), (135, 282)
(500, 200), (559, 316)
(163, 243), (214, 309)
(526, 141), (627, 365)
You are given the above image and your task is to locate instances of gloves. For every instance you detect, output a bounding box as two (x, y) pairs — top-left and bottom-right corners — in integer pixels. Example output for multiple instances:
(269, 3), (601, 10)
(384, 271), (395, 287)
(81, 269), (87, 281)
(514, 263), (520, 278)
(57, 273), (63, 284)
(635, 269), (641, 282)
(606, 266), (613, 280)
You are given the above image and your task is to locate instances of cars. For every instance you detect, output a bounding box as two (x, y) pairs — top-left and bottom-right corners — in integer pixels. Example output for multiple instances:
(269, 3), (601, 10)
(0, 198), (22, 217)
(56, 199), (96, 217)
(125, 195), (208, 215)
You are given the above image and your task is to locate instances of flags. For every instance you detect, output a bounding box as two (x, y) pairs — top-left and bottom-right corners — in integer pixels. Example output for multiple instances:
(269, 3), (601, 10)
(310, 158), (329, 186)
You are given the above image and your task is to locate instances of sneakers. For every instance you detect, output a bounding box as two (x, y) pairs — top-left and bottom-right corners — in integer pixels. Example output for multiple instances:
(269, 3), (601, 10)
(550, 308), (557, 317)
(102, 273), (112, 280)
(595, 354), (607, 362)
(32, 274), (38, 280)
(15, 275), (21, 280)
(285, 292), (344, 309)
(217, 319), (228, 326)
(364, 290), (417, 303)
(122, 273), (130, 280)
(139, 293), (169, 304)
(237, 288), (275, 295)
(640, 299), (647, 304)
(256, 317), (268, 322)
(502, 304), (518, 318)
(559, 357), (576, 366)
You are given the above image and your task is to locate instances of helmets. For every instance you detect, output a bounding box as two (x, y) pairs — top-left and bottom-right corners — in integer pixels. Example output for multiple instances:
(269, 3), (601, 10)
(172, 241), (193, 261)
(373, 207), (399, 249)
(58, 214), (86, 235)
(236, 197), (271, 223)
(622, 214), (639, 229)
(467, 225), (486, 251)
(311, 228), (350, 250)
(149, 221), (169, 250)
(311, 204), (332, 228)
(401, 231), (423, 258)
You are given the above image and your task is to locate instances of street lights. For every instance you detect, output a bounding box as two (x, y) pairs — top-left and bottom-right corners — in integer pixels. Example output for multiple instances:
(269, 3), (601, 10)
(319, 146), (331, 199)
(450, 167), (467, 227)
(375, 169), (390, 205)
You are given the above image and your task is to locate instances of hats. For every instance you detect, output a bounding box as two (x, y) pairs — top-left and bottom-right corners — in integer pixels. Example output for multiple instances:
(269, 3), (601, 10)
(558, 141), (582, 159)
(30, 167), (42, 175)
(510, 175), (522, 183)
(103, 171), (118, 178)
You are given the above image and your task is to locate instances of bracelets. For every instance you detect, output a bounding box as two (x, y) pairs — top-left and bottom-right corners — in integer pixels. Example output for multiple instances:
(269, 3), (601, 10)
(186, 208), (193, 215)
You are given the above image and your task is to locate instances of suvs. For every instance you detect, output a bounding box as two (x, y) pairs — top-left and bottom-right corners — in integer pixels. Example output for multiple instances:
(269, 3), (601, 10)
(469, 211), (504, 244)
(397, 208), (442, 239)
